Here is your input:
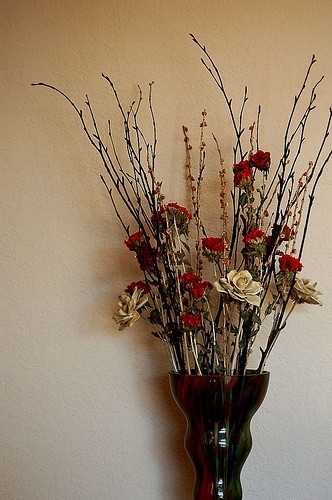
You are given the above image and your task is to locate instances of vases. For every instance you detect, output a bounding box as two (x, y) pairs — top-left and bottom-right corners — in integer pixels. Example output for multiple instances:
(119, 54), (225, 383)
(168, 369), (270, 499)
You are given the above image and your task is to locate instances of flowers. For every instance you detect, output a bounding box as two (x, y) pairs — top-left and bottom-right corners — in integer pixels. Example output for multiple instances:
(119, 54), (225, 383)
(31, 33), (331, 491)
